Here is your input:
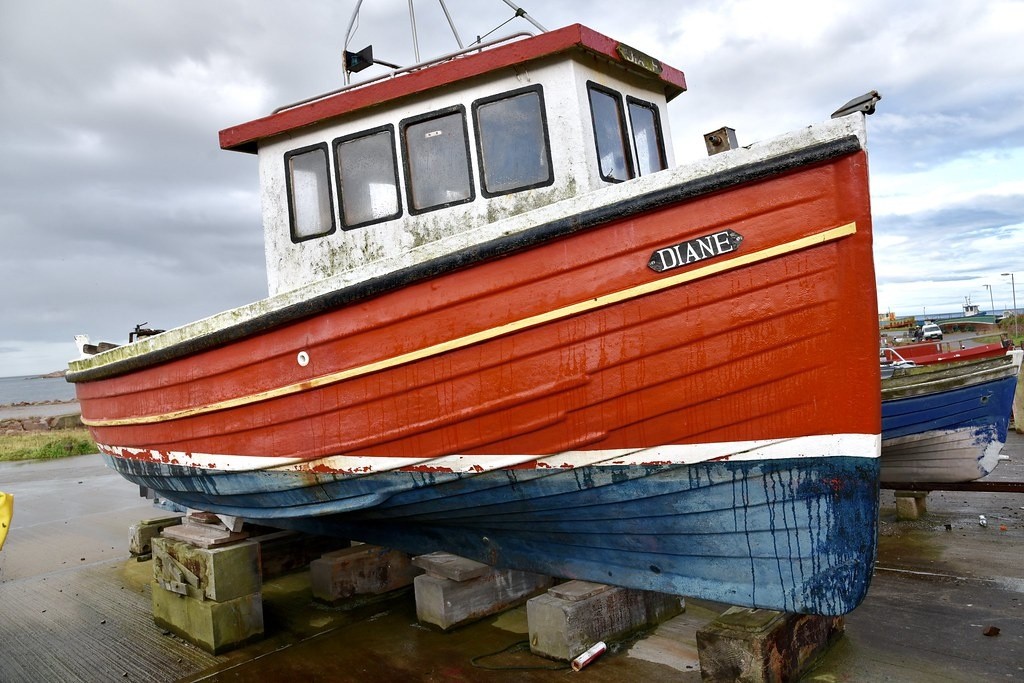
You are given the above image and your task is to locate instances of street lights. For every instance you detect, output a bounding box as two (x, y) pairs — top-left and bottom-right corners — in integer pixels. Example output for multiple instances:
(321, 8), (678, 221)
(982, 283), (995, 324)
(1001, 272), (1018, 339)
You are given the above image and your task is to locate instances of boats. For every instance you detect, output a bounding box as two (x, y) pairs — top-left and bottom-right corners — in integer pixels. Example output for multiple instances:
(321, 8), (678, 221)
(879, 317), (1019, 487)
(57, 20), (888, 623)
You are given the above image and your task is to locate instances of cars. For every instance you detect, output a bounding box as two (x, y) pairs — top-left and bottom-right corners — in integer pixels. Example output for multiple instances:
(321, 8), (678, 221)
(922, 322), (943, 342)
